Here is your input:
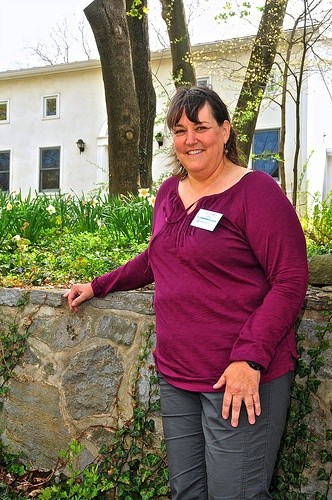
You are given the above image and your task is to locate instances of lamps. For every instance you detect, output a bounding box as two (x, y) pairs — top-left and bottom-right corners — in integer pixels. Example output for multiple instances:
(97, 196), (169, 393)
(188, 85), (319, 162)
(76, 139), (85, 155)
(155, 132), (163, 149)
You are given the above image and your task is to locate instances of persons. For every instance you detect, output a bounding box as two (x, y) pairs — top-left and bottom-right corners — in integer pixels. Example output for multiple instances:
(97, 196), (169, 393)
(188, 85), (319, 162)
(61, 86), (308, 500)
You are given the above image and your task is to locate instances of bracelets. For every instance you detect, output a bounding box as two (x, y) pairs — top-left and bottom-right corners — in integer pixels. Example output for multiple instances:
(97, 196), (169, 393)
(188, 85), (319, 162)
(245, 360), (262, 371)
